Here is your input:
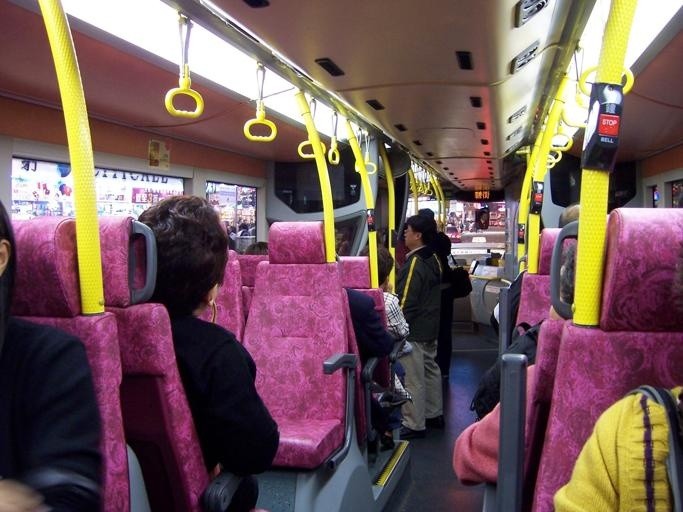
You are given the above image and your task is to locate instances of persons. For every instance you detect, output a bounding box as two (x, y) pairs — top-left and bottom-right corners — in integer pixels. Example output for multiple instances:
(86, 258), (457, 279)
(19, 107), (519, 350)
(360, 245), (413, 449)
(435, 220), (453, 379)
(0, 202), (104, 512)
(220, 218), (256, 252)
(247, 243), (269, 255)
(445, 209), (506, 231)
(137, 196), (280, 476)
(396, 214), (443, 439)
(453, 245), (578, 486)
(489, 203), (580, 337)
(553, 387), (683, 512)
(417, 209), (434, 223)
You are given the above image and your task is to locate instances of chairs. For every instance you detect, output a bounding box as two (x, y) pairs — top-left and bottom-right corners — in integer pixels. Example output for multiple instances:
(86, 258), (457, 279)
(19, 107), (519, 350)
(483, 204), (683, 512)
(10, 213), (410, 511)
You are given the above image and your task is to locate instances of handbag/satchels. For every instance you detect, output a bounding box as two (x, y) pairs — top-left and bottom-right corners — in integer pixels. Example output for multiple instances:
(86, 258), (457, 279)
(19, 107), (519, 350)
(474, 320), (545, 419)
(452, 267), (473, 299)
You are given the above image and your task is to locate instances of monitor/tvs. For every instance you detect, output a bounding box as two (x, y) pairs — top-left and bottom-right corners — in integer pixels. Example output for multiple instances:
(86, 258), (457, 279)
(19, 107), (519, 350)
(292, 162), (349, 210)
(568, 168), (616, 206)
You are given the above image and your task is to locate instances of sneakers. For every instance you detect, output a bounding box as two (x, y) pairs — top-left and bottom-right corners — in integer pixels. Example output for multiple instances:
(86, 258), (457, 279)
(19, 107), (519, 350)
(400, 426), (426, 438)
(426, 415), (445, 428)
